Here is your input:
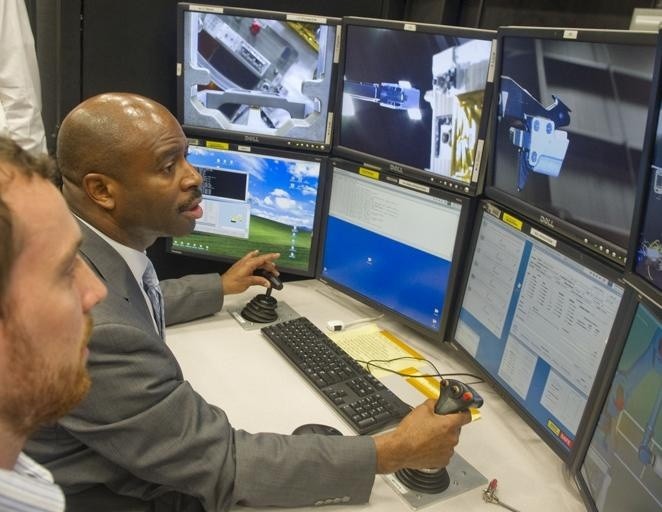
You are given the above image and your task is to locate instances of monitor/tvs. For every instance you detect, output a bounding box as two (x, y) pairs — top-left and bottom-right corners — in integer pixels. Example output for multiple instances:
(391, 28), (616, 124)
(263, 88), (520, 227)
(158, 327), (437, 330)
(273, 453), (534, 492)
(176, 2), (343, 153)
(447, 198), (633, 469)
(314, 158), (477, 344)
(485, 25), (660, 268)
(331, 13), (499, 198)
(165, 137), (329, 279)
(570, 287), (662, 512)
(622, 28), (662, 309)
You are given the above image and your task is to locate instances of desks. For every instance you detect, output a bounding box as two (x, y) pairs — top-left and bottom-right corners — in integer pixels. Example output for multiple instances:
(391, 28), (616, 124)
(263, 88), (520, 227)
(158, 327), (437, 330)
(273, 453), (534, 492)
(166, 279), (588, 510)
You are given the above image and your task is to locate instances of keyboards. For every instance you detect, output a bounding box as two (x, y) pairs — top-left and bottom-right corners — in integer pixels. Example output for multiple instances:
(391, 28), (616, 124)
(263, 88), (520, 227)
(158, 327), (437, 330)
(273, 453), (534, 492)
(261, 316), (413, 435)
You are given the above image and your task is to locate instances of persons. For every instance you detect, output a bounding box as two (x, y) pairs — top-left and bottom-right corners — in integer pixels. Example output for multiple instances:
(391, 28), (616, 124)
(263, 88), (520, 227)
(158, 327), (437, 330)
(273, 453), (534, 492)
(0, 138), (108, 512)
(0, 0), (48, 162)
(21, 93), (473, 511)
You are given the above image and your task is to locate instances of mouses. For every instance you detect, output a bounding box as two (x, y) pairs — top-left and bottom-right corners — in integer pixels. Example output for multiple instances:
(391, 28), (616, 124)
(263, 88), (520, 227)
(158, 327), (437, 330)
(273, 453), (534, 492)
(292, 424), (344, 435)
